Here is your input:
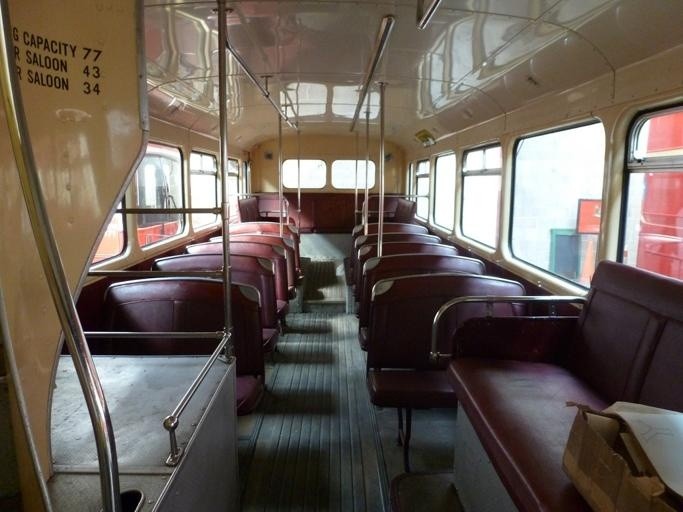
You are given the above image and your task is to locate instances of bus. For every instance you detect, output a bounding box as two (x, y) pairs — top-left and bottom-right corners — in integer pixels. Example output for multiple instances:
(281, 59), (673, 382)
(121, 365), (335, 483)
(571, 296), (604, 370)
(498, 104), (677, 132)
(91, 144), (188, 263)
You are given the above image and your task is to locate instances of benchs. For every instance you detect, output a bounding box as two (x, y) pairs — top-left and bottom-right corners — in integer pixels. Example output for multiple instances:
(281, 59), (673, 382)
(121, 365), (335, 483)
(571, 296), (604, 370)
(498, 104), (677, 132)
(255, 192), (403, 234)
(102, 254), (275, 416)
(430, 260), (683, 512)
(188, 221), (301, 335)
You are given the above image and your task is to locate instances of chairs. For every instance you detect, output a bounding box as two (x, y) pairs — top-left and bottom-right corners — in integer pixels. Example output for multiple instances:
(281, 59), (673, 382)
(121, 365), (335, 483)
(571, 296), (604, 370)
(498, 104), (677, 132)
(352, 223), (525, 454)
